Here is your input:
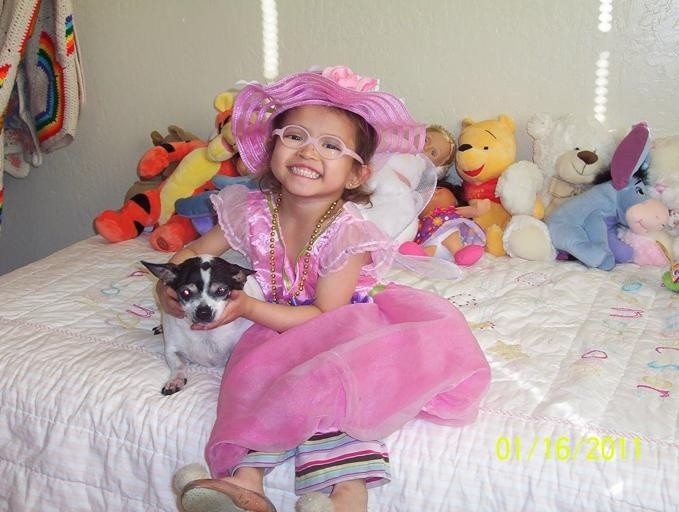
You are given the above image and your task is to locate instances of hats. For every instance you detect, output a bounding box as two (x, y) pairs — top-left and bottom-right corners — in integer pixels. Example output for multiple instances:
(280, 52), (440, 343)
(232, 65), (427, 176)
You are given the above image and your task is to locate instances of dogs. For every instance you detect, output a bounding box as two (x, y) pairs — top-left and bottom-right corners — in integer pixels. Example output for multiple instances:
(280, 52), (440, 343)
(140, 255), (266, 395)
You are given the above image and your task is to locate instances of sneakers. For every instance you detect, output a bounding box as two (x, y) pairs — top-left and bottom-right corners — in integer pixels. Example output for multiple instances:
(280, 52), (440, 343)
(180, 479), (277, 512)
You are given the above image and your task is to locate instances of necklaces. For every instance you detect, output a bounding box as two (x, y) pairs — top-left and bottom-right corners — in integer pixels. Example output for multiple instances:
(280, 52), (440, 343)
(271, 189), (339, 307)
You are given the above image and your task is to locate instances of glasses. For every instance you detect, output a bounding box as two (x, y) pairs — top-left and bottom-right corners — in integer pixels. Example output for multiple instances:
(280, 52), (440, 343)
(271, 125), (365, 164)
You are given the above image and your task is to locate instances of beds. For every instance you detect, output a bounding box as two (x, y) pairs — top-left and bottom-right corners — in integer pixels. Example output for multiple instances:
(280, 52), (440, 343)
(1, 232), (678, 508)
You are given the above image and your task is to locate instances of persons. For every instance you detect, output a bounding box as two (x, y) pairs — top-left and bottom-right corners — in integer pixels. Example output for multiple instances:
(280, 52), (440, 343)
(154, 66), (493, 512)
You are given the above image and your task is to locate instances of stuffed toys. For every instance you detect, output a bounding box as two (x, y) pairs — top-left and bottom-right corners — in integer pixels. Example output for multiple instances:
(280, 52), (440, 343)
(94, 89), (677, 294)
(93, 92), (257, 253)
(360, 114), (679, 293)
